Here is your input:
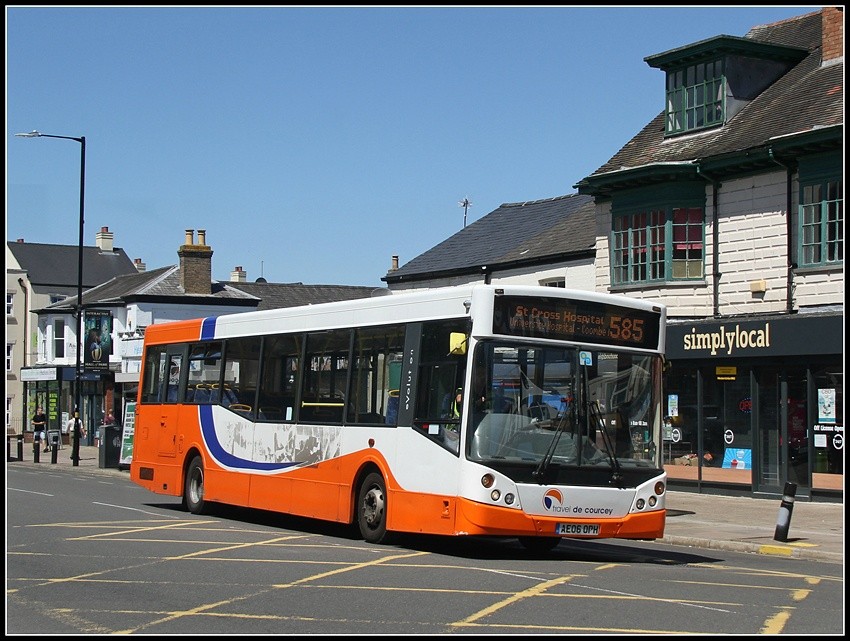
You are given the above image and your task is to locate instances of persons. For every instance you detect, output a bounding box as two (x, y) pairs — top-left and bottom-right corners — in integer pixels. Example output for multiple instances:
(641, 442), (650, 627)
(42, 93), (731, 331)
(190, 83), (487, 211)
(104, 410), (116, 426)
(66, 412), (86, 460)
(30, 407), (48, 452)
(448, 358), (498, 438)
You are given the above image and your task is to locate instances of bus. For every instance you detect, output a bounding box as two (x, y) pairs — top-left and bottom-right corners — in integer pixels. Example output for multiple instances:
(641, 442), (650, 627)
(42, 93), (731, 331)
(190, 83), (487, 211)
(129, 284), (672, 552)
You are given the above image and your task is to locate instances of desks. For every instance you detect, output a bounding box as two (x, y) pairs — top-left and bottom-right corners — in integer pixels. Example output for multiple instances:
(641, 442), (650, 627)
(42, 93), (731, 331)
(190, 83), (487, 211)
(666, 441), (694, 464)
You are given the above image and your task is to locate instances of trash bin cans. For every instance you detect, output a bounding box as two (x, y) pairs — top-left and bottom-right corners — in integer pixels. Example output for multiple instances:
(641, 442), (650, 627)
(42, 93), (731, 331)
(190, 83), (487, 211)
(99, 425), (123, 469)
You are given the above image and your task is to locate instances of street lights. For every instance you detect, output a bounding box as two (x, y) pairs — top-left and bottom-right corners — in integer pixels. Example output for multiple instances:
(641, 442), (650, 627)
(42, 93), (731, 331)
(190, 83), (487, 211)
(15, 130), (86, 464)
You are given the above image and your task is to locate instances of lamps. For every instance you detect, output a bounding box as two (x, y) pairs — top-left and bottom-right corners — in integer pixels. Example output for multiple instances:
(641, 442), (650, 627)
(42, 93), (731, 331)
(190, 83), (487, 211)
(750, 280), (766, 293)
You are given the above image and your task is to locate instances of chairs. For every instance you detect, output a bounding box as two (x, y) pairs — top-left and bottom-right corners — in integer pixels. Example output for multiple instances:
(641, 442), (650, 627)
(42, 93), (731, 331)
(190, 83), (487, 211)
(164, 383), (356, 420)
(387, 388), (400, 427)
